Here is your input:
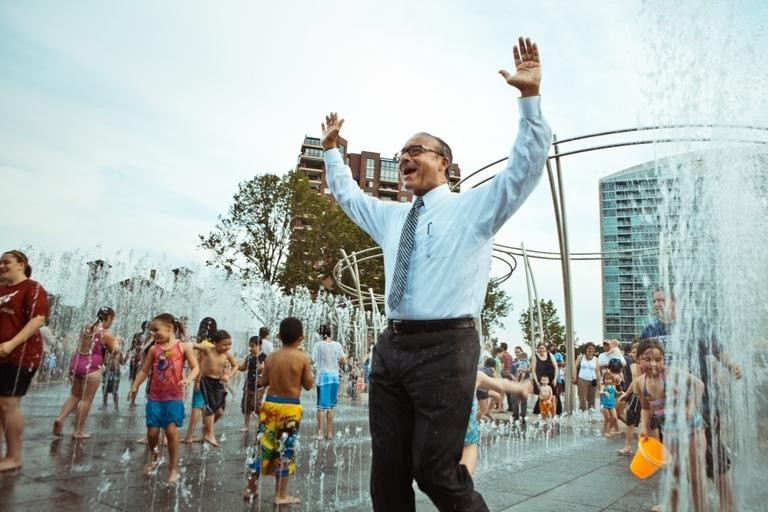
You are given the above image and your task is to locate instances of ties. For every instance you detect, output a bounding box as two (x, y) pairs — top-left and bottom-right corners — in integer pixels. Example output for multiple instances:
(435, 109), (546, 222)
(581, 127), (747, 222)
(387, 197), (425, 312)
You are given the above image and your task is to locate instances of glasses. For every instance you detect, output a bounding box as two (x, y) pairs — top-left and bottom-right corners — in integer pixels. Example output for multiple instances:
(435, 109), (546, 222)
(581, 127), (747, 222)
(395, 145), (445, 163)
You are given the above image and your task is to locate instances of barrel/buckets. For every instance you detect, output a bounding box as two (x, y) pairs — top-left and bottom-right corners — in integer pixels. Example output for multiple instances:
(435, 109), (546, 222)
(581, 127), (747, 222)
(630, 436), (673, 480)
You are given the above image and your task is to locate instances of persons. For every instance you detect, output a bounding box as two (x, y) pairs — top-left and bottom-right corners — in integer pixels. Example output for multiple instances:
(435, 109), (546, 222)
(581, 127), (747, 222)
(618, 343), (641, 456)
(36, 321), (60, 382)
(500, 343), (513, 413)
(548, 343), (564, 394)
(572, 343), (598, 413)
(340, 340), (373, 403)
(180, 331), (237, 444)
(311, 324), (347, 440)
(461, 366), (533, 480)
(189, 330), (238, 447)
(243, 317), (314, 505)
(126, 321), (151, 411)
(125, 313), (200, 482)
(598, 372), (624, 437)
(52, 306), (125, 439)
(636, 339), (707, 512)
(638, 286), (743, 511)
(539, 376), (553, 420)
(513, 352), (529, 382)
(479, 340), (494, 365)
(0, 250), (50, 472)
(475, 357), (502, 423)
(239, 336), (266, 433)
(259, 327), (272, 357)
(510, 346), (531, 424)
(493, 348), (507, 376)
(607, 338), (627, 383)
(98, 336), (130, 413)
(320, 36), (555, 512)
(598, 339), (622, 407)
(532, 342), (558, 415)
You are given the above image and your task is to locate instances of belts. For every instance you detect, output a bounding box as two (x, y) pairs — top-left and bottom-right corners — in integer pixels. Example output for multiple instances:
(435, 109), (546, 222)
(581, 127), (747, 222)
(386, 317), (475, 335)
